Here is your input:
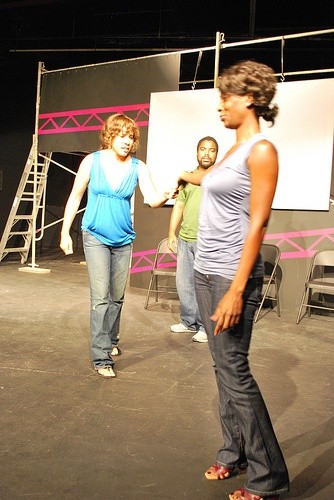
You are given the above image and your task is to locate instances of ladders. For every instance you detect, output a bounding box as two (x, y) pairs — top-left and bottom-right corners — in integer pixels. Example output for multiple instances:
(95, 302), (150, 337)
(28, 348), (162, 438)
(1, 138), (52, 264)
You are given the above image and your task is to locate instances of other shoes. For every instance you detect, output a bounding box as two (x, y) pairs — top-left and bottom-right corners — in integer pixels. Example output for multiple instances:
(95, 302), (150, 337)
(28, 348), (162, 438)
(111, 347), (119, 356)
(95, 366), (115, 378)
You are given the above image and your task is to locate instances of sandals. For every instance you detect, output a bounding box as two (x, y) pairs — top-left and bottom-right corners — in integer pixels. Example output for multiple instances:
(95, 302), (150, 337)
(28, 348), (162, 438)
(205, 463), (248, 480)
(230, 486), (280, 500)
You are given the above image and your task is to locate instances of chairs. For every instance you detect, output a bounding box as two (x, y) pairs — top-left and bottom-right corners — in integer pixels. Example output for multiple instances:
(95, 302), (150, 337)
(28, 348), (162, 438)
(296, 248), (334, 324)
(145, 237), (179, 309)
(254, 243), (280, 322)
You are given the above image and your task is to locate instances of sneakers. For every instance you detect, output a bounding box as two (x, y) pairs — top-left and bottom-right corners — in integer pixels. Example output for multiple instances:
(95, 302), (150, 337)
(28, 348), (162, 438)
(192, 331), (208, 343)
(169, 323), (199, 333)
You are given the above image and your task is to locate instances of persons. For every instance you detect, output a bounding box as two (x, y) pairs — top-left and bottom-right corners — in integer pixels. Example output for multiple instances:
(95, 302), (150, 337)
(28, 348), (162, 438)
(60, 112), (179, 378)
(192, 60), (290, 500)
(168, 136), (218, 343)
